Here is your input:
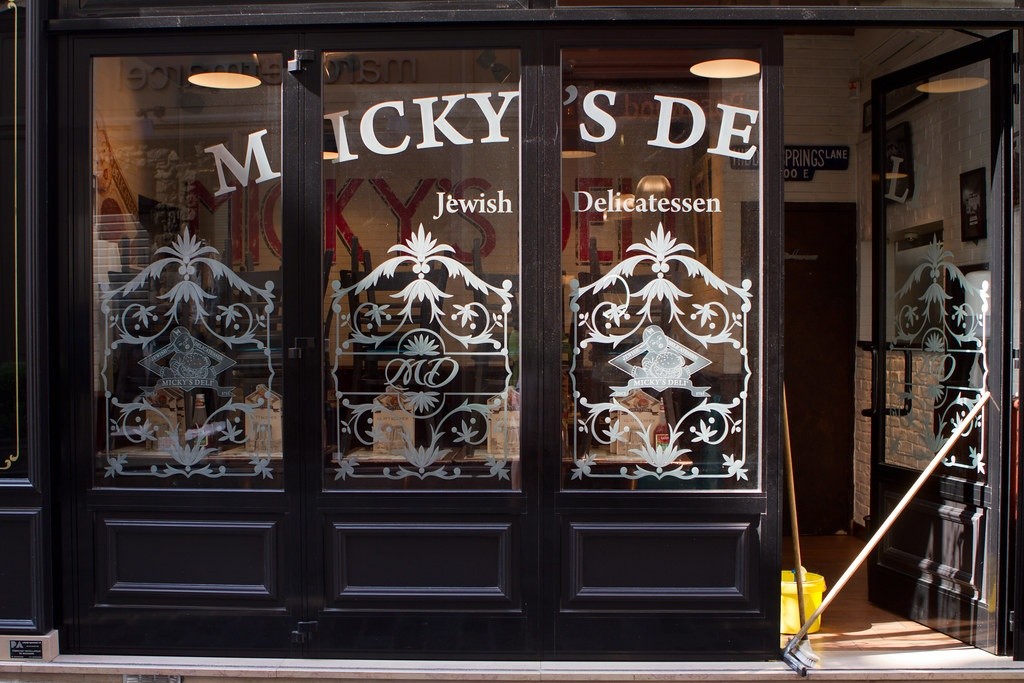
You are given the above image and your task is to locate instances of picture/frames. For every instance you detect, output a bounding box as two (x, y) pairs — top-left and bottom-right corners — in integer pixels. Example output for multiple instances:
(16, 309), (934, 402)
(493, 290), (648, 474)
(862, 78), (929, 135)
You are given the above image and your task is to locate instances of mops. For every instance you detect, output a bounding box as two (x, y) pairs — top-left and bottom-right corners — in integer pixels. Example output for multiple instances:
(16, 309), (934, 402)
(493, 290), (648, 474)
(780, 379), (992, 677)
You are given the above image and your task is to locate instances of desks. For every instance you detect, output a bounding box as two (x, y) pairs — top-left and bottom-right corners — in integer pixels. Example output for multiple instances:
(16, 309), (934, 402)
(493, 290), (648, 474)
(327, 364), (506, 461)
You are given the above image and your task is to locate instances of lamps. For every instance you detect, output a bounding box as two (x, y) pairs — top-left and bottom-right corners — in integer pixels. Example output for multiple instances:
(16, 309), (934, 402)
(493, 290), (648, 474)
(917, 64), (988, 94)
(186, 54), (265, 91)
(689, 49), (763, 80)
(322, 118), (339, 159)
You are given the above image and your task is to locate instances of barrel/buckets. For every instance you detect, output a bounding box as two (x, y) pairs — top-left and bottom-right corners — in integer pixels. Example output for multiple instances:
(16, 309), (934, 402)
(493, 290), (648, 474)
(780, 570), (827, 635)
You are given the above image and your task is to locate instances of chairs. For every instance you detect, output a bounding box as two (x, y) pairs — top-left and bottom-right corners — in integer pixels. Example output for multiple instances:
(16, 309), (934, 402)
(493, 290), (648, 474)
(568, 235), (684, 460)
(108, 234), (336, 451)
(339, 236), (450, 456)
(463, 238), (522, 459)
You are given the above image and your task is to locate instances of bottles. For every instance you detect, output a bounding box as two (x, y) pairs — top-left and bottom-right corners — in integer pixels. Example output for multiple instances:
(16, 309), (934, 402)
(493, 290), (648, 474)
(653, 397), (670, 453)
(192, 393), (209, 448)
